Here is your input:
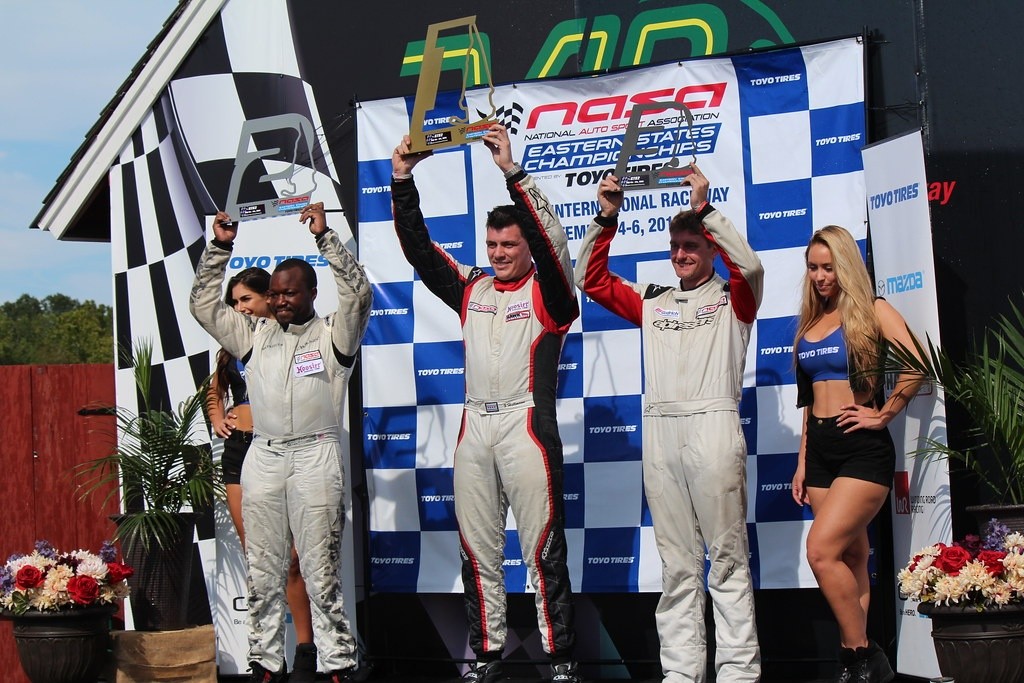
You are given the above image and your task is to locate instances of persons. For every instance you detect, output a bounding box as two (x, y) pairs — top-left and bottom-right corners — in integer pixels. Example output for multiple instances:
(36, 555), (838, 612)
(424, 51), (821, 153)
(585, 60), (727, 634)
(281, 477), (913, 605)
(573, 161), (767, 683)
(189, 202), (375, 682)
(392, 125), (587, 683)
(204, 265), (326, 683)
(789, 225), (929, 683)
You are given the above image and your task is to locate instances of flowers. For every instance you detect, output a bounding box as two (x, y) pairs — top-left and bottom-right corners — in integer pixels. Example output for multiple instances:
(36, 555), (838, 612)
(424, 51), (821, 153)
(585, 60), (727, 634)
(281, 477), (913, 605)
(896, 521), (1024, 605)
(0, 538), (134, 618)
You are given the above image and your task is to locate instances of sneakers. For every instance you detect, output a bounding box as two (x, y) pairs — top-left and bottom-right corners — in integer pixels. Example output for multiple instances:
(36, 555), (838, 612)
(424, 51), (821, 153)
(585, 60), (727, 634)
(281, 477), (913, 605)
(461, 660), (505, 683)
(548, 657), (581, 683)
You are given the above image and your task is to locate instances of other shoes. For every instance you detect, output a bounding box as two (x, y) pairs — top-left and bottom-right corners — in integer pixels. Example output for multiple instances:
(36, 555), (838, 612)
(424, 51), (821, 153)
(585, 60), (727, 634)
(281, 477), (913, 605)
(326, 670), (351, 683)
(247, 660), (285, 683)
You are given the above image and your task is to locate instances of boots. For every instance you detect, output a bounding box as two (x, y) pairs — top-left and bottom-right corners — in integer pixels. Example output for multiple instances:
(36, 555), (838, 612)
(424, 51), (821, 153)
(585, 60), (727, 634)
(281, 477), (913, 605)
(286, 644), (317, 683)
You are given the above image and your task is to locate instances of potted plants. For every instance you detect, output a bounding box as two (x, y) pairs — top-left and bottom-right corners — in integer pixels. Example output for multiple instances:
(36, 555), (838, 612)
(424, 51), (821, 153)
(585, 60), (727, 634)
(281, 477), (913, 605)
(849, 303), (1023, 545)
(72, 333), (222, 629)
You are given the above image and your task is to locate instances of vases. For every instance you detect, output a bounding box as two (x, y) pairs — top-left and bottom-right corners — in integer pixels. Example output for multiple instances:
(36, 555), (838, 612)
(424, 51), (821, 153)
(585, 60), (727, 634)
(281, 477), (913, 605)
(916, 595), (1024, 683)
(7, 600), (121, 682)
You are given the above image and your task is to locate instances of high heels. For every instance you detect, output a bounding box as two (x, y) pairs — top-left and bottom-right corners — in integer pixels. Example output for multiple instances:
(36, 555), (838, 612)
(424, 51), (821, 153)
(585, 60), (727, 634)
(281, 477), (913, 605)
(837, 642), (895, 683)
